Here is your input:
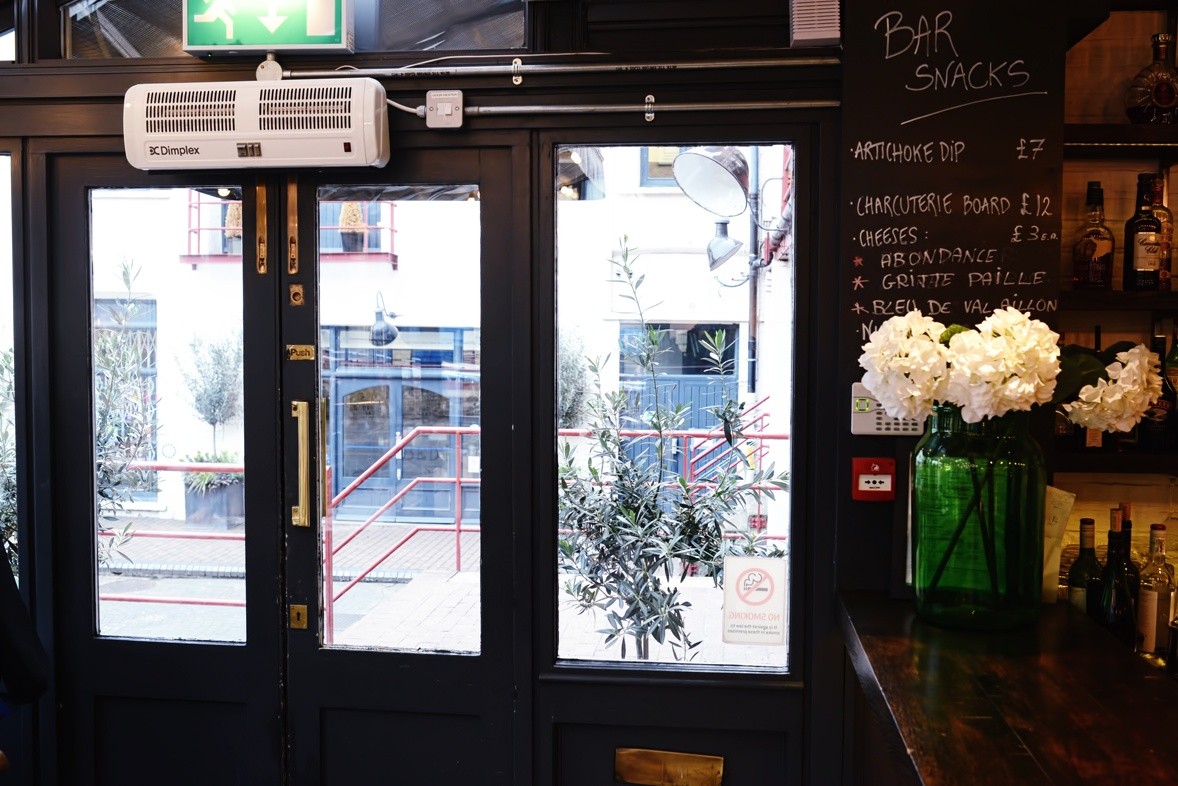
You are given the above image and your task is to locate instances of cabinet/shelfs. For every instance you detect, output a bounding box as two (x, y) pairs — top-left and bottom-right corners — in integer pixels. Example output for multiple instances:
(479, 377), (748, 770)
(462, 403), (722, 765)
(1056, 119), (1178, 481)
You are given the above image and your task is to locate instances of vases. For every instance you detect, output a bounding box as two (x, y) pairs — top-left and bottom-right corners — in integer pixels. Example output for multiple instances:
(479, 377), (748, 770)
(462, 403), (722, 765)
(904, 405), (1047, 635)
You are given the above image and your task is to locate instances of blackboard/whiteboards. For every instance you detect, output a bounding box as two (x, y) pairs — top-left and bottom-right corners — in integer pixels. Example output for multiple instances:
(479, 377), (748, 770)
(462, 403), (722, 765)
(839, 1), (1061, 401)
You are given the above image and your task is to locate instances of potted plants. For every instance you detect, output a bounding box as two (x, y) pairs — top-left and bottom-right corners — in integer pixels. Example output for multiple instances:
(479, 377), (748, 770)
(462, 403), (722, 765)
(340, 201), (364, 254)
(226, 203), (242, 257)
(176, 342), (242, 529)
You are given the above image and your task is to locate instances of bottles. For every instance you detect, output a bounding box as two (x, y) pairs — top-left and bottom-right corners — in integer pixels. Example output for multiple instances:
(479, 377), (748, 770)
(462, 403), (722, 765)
(1152, 172), (1173, 290)
(1124, 173), (1163, 290)
(1136, 334), (1178, 454)
(1067, 501), (1178, 666)
(1124, 33), (1178, 125)
(906, 400), (1048, 642)
(1071, 180), (1117, 291)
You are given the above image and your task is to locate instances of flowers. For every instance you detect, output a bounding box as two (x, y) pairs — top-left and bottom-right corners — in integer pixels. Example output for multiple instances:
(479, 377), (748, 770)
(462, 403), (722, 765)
(859, 307), (1166, 444)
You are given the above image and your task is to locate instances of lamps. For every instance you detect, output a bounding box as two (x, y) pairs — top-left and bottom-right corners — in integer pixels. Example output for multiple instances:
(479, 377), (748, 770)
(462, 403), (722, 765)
(371, 284), (402, 347)
(705, 177), (785, 270)
(670, 144), (789, 237)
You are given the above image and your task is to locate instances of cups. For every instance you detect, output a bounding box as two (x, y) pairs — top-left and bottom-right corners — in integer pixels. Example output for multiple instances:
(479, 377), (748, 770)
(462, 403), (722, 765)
(1058, 544), (1176, 604)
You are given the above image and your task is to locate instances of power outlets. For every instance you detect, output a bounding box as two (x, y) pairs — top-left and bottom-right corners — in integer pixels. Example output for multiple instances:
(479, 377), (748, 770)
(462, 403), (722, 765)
(425, 87), (464, 131)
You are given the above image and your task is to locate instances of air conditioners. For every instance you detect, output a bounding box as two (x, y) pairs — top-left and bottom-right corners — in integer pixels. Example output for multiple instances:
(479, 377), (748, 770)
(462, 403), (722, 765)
(123, 81), (389, 171)
